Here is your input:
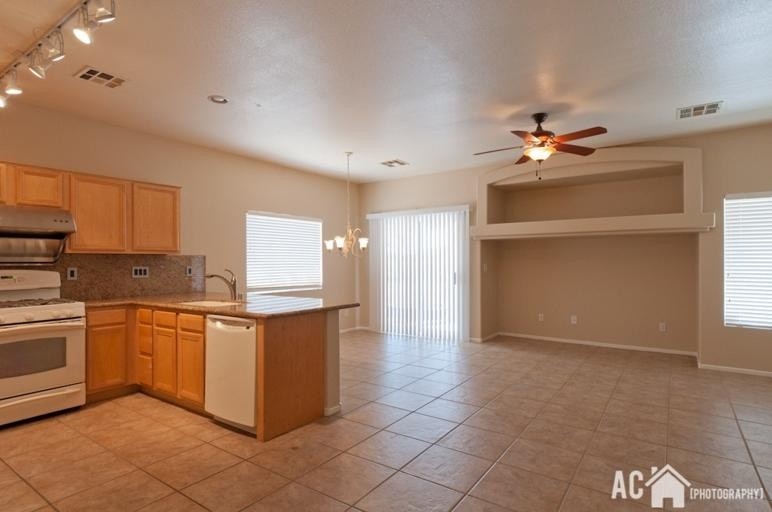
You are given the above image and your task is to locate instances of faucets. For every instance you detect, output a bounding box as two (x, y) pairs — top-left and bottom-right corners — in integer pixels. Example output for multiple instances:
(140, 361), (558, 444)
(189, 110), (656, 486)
(204, 268), (237, 301)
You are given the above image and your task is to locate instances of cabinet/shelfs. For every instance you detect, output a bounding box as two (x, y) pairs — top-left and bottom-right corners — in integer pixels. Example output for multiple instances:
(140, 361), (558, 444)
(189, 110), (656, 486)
(67, 173), (128, 253)
(0, 161), (65, 211)
(88, 302), (130, 406)
(130, 182), (180, 254)
(132, 302), (205, 405)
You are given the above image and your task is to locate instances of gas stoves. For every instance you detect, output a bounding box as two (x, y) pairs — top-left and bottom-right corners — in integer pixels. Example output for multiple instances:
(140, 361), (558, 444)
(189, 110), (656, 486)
(0, 298), (86, 326)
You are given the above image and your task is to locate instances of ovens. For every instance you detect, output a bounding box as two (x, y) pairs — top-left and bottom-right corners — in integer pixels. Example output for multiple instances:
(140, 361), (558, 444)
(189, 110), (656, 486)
(1, 317), (87, 426)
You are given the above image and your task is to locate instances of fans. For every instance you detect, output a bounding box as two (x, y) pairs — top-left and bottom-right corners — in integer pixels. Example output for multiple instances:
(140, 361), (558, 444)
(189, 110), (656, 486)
(471, 112), (608, 164)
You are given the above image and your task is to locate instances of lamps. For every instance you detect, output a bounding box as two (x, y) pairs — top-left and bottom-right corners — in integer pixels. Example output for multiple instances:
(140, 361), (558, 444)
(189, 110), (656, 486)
(322, 151), (370, 260)
(523, 145), (556, 182)
(1, 1), (118, 111)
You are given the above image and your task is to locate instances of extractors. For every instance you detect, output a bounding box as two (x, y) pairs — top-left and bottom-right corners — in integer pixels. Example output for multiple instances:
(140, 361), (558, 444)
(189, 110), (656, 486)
(1, 202), (77, 265)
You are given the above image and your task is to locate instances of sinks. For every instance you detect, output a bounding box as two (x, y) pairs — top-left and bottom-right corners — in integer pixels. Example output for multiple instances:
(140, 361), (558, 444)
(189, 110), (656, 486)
(168, 299), (253, 307)
